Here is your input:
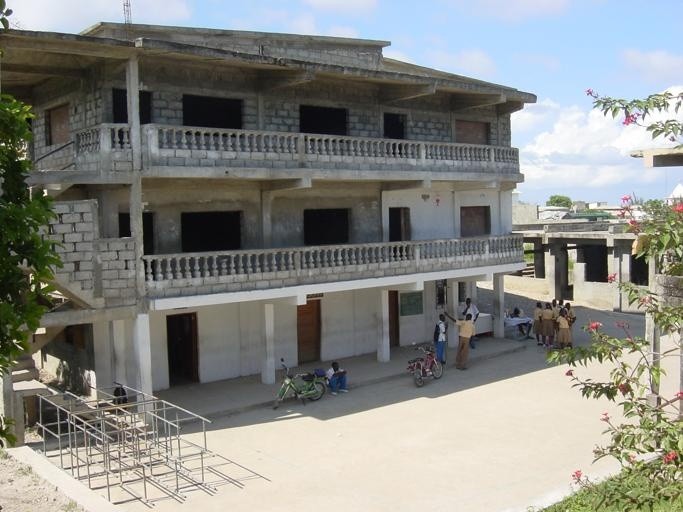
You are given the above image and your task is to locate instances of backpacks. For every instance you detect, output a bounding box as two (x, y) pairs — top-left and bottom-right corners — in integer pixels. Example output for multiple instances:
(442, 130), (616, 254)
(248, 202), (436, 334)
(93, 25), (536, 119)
(434, 321), (444, 341)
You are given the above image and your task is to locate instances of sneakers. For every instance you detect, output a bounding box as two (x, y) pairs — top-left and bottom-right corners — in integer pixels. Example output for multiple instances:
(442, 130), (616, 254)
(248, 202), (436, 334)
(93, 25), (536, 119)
(537, 343), (573, 349)
(332, 389), (349, 395)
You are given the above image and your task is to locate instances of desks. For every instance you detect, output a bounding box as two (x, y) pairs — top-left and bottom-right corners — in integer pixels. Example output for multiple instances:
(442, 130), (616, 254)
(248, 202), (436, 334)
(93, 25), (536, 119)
(503, 317), (531, 341)
(474, 313), (493, 336)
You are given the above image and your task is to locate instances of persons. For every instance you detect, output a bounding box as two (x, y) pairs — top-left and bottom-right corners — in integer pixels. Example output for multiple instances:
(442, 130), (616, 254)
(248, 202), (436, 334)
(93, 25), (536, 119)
(512, 306), (534, 339)
(532, 298), (576, 349)
(433, 314), (447, 364)
(443, 311), (475, 369)
(461, 297), (480, 349)
(324, 361), (349, 396)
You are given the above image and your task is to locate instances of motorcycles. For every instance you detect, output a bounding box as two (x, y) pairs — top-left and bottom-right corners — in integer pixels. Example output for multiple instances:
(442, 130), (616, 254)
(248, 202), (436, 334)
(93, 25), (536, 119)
(407, 346), (442, 387)
(273, 358), (328, 405)
